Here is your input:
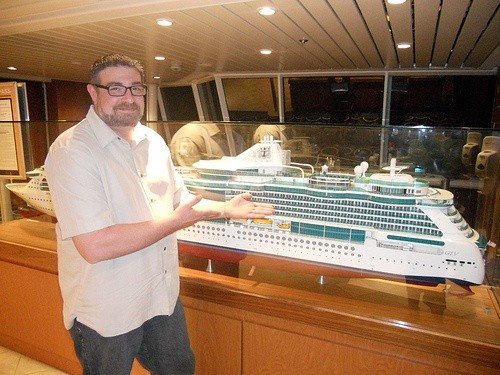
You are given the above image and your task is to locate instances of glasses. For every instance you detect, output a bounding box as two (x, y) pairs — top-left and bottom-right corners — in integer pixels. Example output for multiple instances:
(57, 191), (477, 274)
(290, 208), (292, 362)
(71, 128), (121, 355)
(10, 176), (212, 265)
(94, 83), (147, 96)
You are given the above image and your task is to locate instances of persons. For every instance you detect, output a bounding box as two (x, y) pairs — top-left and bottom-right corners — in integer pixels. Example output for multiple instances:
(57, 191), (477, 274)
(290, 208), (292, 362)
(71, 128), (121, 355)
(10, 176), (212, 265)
(254, 124), (296, 150)
(170, 121), (248, 280)
(406, 131), (470, 322)
(44, 53), (275, 375)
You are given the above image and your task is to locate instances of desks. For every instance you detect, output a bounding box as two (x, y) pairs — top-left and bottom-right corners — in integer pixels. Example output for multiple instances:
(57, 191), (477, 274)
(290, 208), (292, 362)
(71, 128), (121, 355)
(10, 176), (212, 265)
(0, 214), (500, 375)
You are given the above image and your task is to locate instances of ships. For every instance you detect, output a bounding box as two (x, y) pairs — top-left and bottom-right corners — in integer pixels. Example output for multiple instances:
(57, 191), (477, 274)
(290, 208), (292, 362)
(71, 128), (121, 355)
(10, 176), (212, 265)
(4, 134), (488, 286)
